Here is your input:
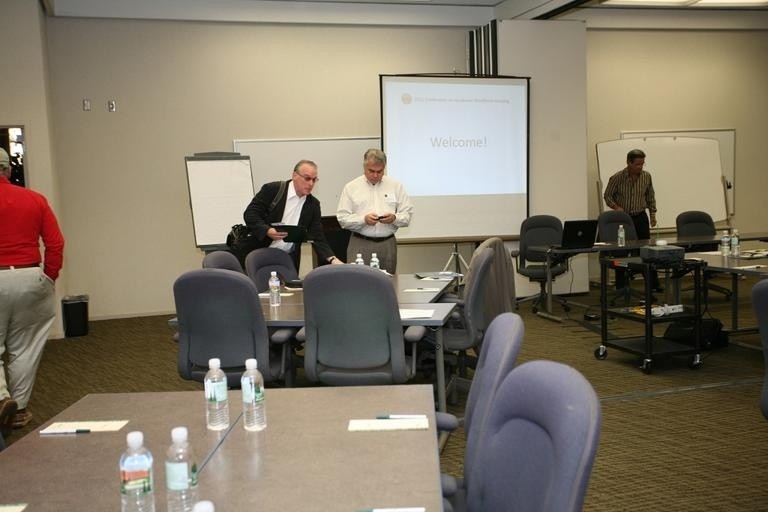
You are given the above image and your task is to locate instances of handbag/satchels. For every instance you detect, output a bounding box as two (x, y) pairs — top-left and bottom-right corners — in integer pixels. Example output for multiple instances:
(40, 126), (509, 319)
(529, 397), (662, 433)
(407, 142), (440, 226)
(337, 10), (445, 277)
(227, 223), (256, 254)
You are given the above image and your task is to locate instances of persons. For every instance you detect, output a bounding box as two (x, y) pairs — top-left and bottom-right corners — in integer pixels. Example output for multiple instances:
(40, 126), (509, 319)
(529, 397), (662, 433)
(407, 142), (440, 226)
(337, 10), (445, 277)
(243, 159), (344, 275)
(0, 148), (65, 440)
(335, 148), (414, 275)
(604, 150), (666, 293)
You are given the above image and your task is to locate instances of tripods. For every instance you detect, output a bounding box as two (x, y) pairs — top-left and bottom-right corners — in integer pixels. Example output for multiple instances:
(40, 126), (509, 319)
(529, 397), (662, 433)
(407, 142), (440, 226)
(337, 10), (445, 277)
(440, 242), (468, 293)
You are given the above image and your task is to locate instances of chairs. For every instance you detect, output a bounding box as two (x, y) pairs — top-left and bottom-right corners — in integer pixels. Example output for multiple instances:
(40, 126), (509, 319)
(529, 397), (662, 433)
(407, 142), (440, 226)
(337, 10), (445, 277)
(597, 212), (659, 306)
(295, 264), (426, 386)
(442, 359), (600, 512)
(510, 214), (573, 313)
(423, 237), (503, 377)
(203, 251), (244, 274)
(751, 279), (768, 420)
(669, 211), (731, 303)
(245, 247), (301, 296)
(171, 269), (297, 387)
(406, 248), (496, 411)
(436, 312), (524, 497)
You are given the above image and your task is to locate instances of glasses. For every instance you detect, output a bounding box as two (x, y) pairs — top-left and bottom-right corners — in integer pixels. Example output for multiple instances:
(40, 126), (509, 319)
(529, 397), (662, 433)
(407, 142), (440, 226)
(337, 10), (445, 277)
(295, 170), (319, 183)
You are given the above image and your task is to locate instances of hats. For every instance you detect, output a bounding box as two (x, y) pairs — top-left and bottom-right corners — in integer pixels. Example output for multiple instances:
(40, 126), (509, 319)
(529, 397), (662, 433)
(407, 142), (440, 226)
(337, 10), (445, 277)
(0, 148), (11, 174)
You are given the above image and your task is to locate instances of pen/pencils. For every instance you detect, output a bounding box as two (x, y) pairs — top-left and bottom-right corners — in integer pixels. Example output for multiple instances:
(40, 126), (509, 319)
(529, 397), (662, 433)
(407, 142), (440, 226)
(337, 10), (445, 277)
(359, 507), (426, 512)
(377, 414), (428, 419)
(417, 287), (423, 290)
(40, 429), (90, 433)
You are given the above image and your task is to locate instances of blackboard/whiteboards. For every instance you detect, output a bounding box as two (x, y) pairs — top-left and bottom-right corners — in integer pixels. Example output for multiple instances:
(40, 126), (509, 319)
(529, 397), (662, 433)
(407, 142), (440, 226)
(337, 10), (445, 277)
(233, 136), (381, 217)
(596, 129), (736, 230)
(185, 156), (255, 248)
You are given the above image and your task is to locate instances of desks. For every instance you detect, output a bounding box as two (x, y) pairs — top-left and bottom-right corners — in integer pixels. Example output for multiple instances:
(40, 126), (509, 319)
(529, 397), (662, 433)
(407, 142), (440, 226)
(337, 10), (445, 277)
(165, 383), (444, 512)
(168, 302), (457, 412)
(684, 248), (768, 352)
(528, 233), (768, 322)
(257, 273), (460, 304)
(0, 393), (241, 512)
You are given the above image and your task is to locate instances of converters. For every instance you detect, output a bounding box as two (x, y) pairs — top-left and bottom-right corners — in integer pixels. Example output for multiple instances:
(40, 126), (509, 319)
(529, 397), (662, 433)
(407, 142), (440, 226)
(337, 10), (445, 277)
(584, 313), (601, 320)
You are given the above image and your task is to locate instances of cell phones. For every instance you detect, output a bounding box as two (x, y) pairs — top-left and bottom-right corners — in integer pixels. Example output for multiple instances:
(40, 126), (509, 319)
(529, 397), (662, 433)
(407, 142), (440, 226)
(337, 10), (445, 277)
(374, 216), (386, 220)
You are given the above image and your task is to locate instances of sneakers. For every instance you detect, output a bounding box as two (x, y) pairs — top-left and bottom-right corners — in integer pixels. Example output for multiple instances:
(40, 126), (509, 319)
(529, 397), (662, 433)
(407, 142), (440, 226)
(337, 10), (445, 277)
(0, 397), (34, 436)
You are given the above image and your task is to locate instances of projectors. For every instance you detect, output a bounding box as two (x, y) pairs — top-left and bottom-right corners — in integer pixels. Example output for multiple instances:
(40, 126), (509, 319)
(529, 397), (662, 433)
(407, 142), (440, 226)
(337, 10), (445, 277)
(640, 245), (685, 263)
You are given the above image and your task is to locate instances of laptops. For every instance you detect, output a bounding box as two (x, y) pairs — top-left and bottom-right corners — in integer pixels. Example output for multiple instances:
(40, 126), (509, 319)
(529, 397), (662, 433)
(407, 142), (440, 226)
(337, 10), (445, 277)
(549, 220), (599, 249)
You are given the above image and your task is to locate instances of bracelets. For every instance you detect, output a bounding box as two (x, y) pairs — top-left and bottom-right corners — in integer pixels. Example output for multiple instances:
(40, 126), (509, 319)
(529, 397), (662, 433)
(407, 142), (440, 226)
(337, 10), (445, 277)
(330, 257), (337, 263)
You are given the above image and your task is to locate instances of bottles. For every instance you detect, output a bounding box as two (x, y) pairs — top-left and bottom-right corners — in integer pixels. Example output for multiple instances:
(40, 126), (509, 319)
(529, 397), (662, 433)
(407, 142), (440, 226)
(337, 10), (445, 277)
(720, 230), (742, 258)
(355, 253), (380, 270)
(163, 426), (216, 512)
(116, 431), (157, 511)
(617, 225), (626, 246)
(267, 271), (282, 307)
(203, 357), (231, 431)
(239, 357), (269, 433)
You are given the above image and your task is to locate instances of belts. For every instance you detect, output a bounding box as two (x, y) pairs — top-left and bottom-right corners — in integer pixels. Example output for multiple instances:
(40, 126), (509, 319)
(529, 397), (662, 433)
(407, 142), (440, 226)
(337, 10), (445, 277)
(351, 232), (395, 243)
(0, 263), (40, 271)
